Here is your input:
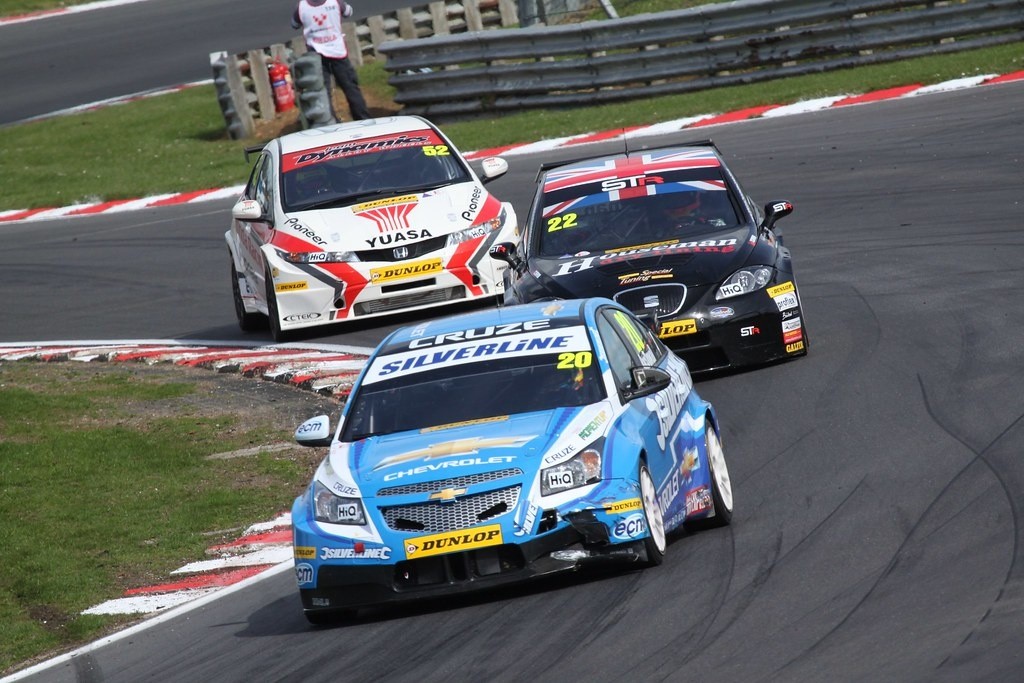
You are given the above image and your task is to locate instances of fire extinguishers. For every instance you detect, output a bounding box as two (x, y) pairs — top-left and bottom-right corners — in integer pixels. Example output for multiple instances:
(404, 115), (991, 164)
(267, 55), (295, 111)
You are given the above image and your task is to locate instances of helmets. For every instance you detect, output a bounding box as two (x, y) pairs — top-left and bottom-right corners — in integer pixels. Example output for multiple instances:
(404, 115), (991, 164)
(533, 364), (583, 404)
(660, 192), (701, 219)
(294, 167), (329, 197)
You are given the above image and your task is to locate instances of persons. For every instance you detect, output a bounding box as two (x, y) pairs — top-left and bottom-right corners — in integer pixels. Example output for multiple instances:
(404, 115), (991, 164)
(533, 363), (586, 409)
(291, 162), (345, 200)
(290, 0), (373, 123)
(655, 187), (727, 235)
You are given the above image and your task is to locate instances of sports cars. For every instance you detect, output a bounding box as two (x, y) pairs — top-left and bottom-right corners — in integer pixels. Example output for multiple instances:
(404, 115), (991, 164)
(488, 138), (810, 382)
(291, 298), (735, 623)
(224, 115), (522, 344)
